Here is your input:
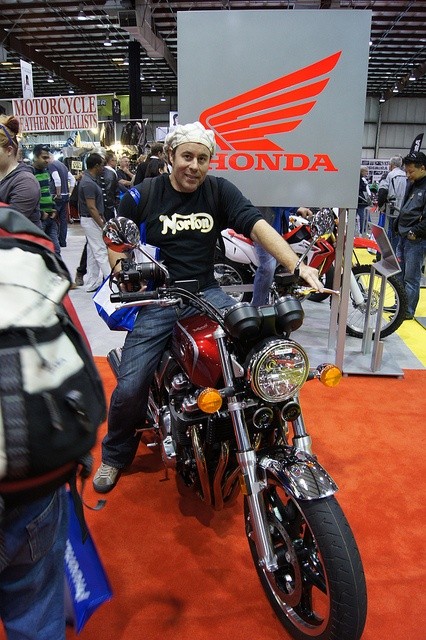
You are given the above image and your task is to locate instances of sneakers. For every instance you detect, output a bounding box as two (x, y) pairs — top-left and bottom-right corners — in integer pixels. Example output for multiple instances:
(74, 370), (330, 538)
(93, 462), (119, 491)
(59, 242), (65, 246)
(75, 273), (83, 285)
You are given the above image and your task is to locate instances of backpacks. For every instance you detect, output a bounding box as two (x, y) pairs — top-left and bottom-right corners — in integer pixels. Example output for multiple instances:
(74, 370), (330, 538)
(0, 204), (106, 512)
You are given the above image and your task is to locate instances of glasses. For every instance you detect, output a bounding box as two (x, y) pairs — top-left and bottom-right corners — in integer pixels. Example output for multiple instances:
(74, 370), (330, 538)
(407, 153), (414, 158)
(0, 124), (13, 145)
(112, 159), (117, 161)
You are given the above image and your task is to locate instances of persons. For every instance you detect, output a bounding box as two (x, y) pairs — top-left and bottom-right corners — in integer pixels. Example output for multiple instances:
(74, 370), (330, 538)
(2, 117), (43, 235)
(378, 155), (408, 266)
(383, 150), (426, 321)
(91, 127), (324, 494)
(1, 478), (69, 639)
(116, 143), (167, 190)
(359, 165), (372, 235)
(31, 144), (77, 256)
(73, 149), (116, 292)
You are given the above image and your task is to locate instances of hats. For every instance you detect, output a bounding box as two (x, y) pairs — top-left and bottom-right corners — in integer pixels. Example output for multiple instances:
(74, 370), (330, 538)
(164, 121), (214, 154)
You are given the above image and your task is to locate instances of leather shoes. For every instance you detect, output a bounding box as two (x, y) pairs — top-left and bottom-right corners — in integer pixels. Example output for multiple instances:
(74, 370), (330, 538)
(86, 282), (99, 291)
(384, 305), (397, 311)
(390, 313), (414, 320)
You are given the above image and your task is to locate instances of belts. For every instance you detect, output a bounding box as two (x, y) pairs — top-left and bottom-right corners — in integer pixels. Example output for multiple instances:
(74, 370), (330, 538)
(80, 214), (102, 217)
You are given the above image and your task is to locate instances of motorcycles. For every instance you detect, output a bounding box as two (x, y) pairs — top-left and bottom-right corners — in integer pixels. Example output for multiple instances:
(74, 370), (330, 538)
(103, 207), (368, 640)
(213, 208), (407, 340)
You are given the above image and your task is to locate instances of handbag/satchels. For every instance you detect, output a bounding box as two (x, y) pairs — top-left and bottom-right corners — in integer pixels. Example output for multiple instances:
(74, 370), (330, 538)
(92, 188), (160, 331)
